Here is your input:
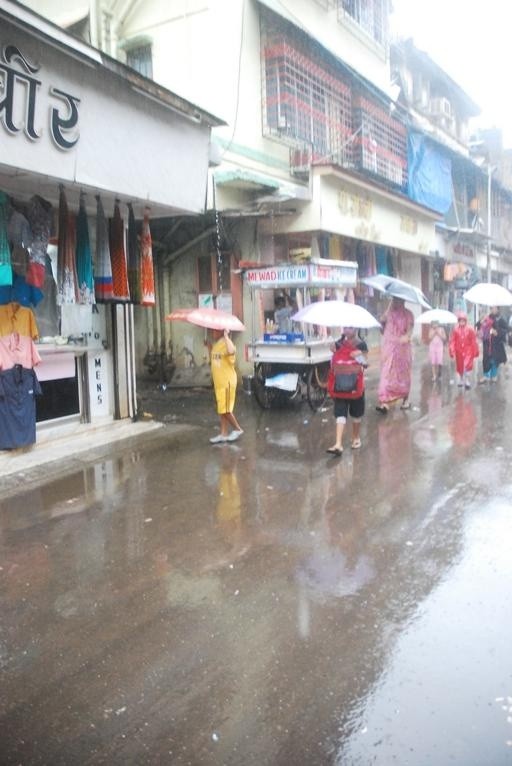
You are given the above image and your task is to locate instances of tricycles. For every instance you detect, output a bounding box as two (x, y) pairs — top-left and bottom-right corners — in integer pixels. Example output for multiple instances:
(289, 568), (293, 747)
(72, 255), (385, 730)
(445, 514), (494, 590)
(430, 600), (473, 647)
(244, 257), (369, 414)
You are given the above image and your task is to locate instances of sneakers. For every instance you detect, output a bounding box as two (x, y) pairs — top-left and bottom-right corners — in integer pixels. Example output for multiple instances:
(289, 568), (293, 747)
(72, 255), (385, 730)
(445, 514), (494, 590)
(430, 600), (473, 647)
(209, 435), (228, 443)
(326, 447), (343, 455)
(227, 429), (244, 441)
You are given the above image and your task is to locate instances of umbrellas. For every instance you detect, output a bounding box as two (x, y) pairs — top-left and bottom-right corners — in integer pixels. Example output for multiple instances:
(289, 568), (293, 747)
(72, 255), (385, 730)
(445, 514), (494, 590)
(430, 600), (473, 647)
(289, 300), (383, 330)
(164, 307), (248, 332)
(358, 272), (435, 317)
(416, 308), (459, 324)
(463, 281), (512, 309)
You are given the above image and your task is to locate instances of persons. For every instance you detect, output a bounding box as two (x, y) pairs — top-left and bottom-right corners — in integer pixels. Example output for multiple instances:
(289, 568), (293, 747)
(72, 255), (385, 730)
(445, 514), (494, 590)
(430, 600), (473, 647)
(375, 296), (415, 412)
(448, 312), (479, 390)
(204, 328), (244, 443)
(428, 320), (447, 381)
(270, 294), (293, 333)
(325, 326), (370, 454)
(474, 305), (509, 385)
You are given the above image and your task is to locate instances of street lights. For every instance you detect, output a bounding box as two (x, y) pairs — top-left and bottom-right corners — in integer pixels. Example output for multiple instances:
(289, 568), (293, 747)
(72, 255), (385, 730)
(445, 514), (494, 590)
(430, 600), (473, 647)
(486, 164), (498, 317)
